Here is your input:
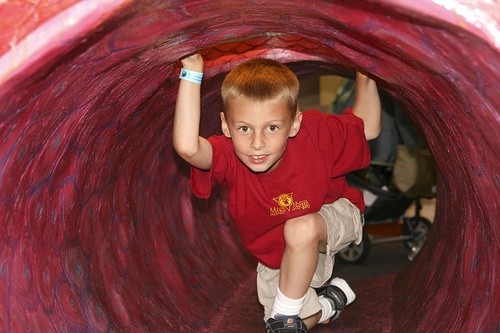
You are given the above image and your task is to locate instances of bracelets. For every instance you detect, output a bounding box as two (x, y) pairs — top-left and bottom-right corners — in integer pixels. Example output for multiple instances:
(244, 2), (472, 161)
(177, 68), (203, 85)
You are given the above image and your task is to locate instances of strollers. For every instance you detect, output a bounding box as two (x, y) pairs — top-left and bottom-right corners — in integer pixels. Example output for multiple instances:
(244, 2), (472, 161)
(333, 78), (437, 265)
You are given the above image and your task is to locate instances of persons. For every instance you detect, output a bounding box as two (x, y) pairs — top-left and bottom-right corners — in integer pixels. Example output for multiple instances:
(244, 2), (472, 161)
(173, 51), (381, 333)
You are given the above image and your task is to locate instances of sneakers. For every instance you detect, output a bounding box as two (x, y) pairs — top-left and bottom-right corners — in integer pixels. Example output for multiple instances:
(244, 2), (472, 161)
(317, 277), (356, 327)
(266, 313), (308, 333)
